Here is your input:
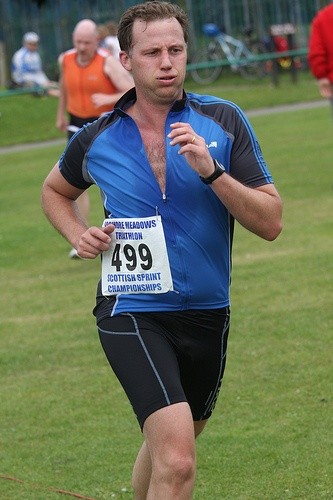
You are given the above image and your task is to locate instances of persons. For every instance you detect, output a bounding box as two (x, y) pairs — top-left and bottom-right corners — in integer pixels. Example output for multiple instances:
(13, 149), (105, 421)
(307, 0), (333, 103)
(41, 2), (284, 500)
(11, 32), (61, 97)
(96, 22), (122, 62)
(56, 18), (135, 260)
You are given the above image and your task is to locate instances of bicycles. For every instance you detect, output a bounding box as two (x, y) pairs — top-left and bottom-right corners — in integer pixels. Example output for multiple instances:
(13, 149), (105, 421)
(189, 24), (269, 85)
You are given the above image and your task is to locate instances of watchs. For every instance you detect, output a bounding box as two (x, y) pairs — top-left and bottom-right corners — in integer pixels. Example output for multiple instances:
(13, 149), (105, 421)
(198, 158), (226, 186)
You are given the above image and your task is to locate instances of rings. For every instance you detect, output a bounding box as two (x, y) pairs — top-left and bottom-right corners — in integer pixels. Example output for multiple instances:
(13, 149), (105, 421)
(191, 135), (195, 144)
